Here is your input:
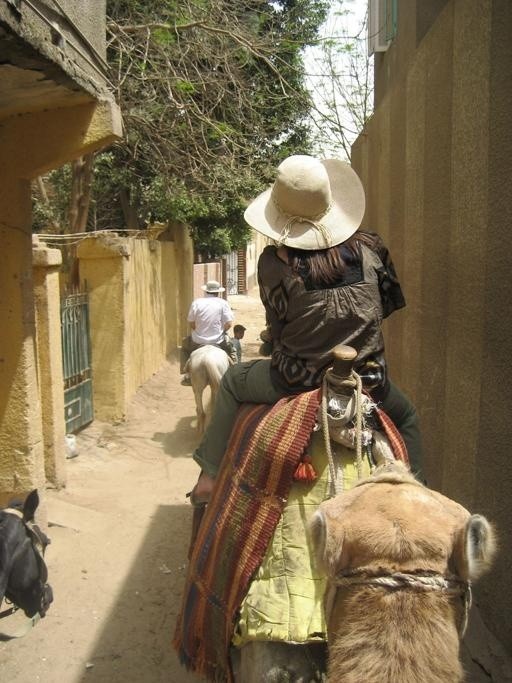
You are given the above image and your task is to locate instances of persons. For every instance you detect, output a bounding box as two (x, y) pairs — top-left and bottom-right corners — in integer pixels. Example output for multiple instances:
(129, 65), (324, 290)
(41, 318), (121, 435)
(180, 279), (237, 386)
(190, 154), (424, 506)
(230, 323), (246, 362)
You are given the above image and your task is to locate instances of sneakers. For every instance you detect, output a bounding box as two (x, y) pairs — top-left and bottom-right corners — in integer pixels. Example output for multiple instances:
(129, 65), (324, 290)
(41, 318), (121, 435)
(181, 378), (192, 386)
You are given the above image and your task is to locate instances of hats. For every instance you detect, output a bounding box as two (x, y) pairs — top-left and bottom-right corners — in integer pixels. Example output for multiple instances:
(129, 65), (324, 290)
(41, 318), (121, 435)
(244, 154), (366, 250)
(201, 279), (225, 292)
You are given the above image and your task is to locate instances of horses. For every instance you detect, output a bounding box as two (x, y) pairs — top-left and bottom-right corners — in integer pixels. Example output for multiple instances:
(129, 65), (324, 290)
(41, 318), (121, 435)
(0, 488), (54, 619)
(189, 344), (234, 437)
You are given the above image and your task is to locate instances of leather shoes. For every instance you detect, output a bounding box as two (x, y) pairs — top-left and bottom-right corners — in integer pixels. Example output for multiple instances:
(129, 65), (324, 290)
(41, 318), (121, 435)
(189, 490), (210, 504)
